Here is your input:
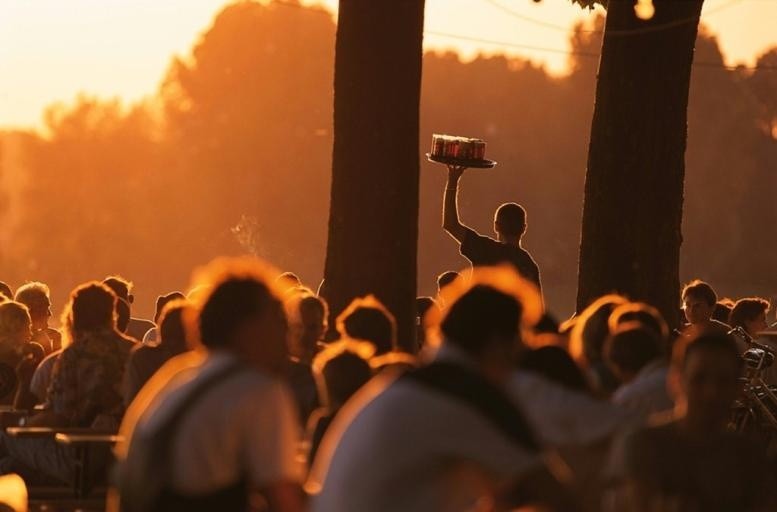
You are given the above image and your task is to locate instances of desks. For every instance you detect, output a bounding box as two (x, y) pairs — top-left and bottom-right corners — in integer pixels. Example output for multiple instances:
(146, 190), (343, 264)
(0, 404), (125, 512)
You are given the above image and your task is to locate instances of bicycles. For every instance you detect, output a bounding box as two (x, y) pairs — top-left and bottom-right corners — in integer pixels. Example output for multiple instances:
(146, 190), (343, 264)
(670, 323), (777, 441)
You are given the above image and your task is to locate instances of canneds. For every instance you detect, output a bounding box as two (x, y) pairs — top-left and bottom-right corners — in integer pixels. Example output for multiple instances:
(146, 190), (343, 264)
(431, 134), (486, 162)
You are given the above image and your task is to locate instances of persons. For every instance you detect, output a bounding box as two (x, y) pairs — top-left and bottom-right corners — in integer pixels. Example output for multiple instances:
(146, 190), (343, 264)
(0, 269), (776, 511)
(444, 165), (541, 289)
